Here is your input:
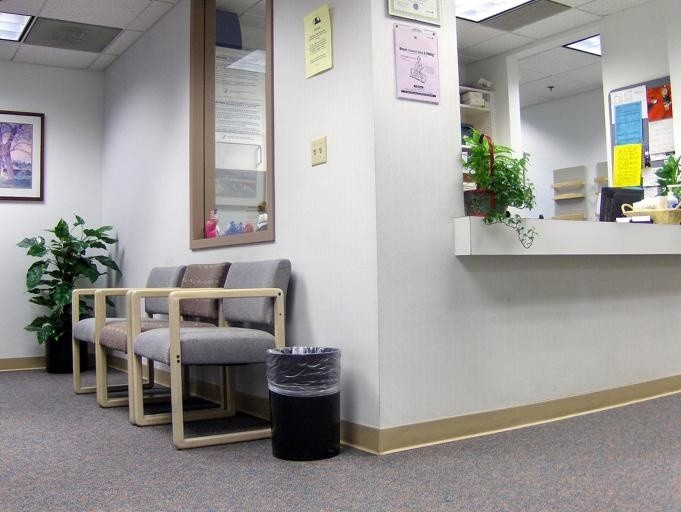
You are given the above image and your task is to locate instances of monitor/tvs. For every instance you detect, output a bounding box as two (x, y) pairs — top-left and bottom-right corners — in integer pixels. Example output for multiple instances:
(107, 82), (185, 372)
(600, 186), (644, 222)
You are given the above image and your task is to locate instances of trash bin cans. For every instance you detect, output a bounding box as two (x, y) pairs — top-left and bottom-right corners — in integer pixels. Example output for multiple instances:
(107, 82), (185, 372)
(265, 346), (341, 461)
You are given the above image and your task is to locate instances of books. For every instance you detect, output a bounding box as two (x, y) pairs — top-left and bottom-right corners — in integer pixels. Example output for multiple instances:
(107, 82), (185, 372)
(460, 90), (485, 107)
(460, 122), (480, 191)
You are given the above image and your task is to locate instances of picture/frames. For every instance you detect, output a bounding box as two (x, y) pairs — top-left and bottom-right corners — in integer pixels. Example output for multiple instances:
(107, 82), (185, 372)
(388, 0), (442, 27)
(214, 168), (266, 208)
(0, 110), (45, 201)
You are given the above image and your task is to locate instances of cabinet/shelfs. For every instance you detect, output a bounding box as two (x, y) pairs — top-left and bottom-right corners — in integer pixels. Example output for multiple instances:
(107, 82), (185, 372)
(550, 180), (586, 221)
(459, 86), (495, 191)
(593, 178), (609, 216)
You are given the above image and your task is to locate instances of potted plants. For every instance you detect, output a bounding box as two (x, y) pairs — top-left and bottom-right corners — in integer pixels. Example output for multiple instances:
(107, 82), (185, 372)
(15, 212), (123, 374)
(460, 126), (541, 248)
(654, 154), (681, 211)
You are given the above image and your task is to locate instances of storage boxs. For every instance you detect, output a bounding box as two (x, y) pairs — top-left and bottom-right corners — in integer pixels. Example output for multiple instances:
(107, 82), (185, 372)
(461, 91), (482, 101)
(464, 99), (485, 107)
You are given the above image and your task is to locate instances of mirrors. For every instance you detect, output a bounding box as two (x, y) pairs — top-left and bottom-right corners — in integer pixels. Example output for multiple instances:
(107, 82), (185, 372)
(187, 1), (275, 250)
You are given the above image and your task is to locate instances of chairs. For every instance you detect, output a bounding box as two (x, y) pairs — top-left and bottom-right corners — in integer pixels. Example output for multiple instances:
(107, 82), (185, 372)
(97, 261), (237, 428)
(70, 265), (190, 409)
(129, 258), (290, 451)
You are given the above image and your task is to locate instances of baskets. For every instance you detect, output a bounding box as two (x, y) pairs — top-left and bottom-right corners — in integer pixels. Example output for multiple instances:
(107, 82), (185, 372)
(621, 202), (681, 225)
